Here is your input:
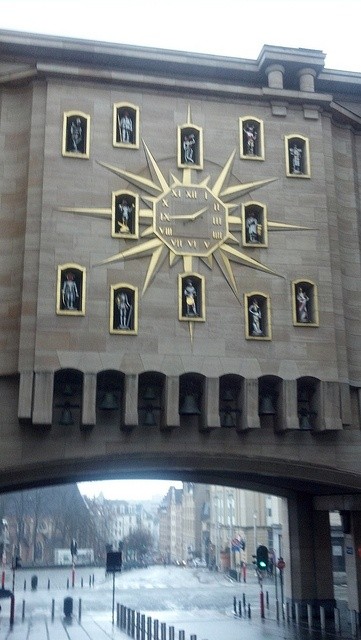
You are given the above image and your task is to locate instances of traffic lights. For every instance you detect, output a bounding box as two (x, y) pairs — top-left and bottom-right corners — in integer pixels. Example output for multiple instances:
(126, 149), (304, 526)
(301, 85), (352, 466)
(258, 545), (269, 570)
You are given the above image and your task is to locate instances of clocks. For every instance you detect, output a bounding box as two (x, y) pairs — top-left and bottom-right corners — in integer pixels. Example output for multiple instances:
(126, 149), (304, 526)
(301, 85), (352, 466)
(149, 183), (231, 257)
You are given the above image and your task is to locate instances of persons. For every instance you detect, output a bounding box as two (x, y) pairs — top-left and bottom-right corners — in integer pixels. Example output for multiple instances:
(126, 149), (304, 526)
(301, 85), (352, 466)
(288, 144), (304, 175)
(249, 298), (265, 337)
(118, 198), (135, 229)
(70, 118), (84, 151)
(243, 122), (259, 155)
(118, 110), (133, 142)
(245, 212), (260, 244)
(181, 277), (198, 316)
(181, 133), (197, 163)
(60, 270), (80, 311)
(294, 284), (312, 323)
(115, 289), (133, 328)
